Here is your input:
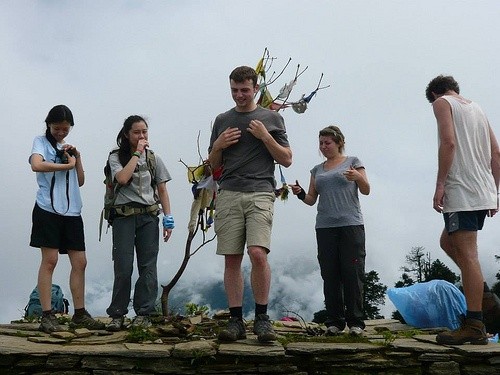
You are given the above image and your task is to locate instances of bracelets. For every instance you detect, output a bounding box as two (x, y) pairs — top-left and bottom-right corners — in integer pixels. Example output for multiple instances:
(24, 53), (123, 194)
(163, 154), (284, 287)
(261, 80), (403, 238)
(162, 216), (174, 228)
(75, 153), (81, 158)
(134, 149), (143, 158)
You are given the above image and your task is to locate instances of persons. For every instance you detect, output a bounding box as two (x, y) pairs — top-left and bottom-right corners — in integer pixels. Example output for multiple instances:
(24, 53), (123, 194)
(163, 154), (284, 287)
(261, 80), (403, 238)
(426, 75), (500, 346)
(289, 126), (370, 337)
(105, 115), (174, 331)
(28, 105), (104, 333)
(208, 66), (292, 343)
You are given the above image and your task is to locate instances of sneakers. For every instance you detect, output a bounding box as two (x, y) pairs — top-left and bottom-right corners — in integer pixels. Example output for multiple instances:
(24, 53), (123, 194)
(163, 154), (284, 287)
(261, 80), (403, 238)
(69, 309), (105, 331)
(218, 315), (247, 342)
(482, 293), (500, 319)
(106, 314), (126, 331)
(253, 314), (277, 342)
(349, 326), (364, 337)
(132, 315), (146, 327)
(435, 318), (489, 345)
(324, 326), (345, 336)
(38, 314), (64, 334)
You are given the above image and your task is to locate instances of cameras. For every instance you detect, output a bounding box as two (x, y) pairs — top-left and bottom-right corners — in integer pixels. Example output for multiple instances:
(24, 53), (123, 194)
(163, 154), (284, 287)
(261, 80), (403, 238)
(56, 148), (73, 164)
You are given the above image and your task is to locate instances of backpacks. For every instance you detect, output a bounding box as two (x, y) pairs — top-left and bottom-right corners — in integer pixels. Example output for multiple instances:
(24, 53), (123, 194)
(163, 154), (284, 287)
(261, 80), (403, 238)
(24, 284), (70, 319)
(104, 148), (159, 221)
